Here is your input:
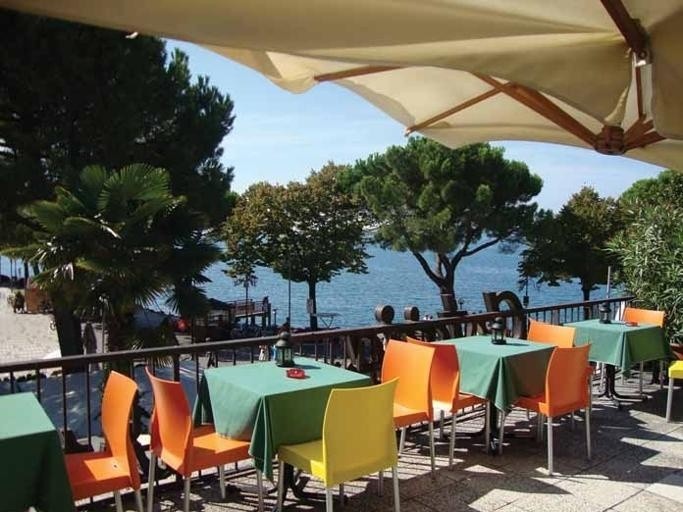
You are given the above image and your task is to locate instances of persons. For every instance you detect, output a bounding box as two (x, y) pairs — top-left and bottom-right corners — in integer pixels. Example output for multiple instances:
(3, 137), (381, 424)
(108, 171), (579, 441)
(2, 290), (483, 379)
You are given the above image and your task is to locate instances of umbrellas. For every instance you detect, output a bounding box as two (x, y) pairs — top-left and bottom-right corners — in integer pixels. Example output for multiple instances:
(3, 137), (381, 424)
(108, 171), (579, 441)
(0, 0), (682, 174)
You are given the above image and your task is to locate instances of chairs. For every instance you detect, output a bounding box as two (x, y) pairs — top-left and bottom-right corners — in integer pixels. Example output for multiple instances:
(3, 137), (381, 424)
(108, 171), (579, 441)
(499, 340), (596, 478)
(664, 361), (681, 423)
(529, 320), (594, 432)
(610, 307), (668, 396)
(146, 367), (266, 512)
(65, 371), (142, 511)
(405, 336), (490, 468)
(373, 339), (439, 491)
(272, 376), (403, 511)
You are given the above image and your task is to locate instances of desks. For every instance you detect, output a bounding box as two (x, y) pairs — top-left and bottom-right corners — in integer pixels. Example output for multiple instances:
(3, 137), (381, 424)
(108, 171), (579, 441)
(2, 391), (82, 510)
(431, 336), (558, 455)
(203, 357), (371, 510)
(564, 319), (663, 409)
